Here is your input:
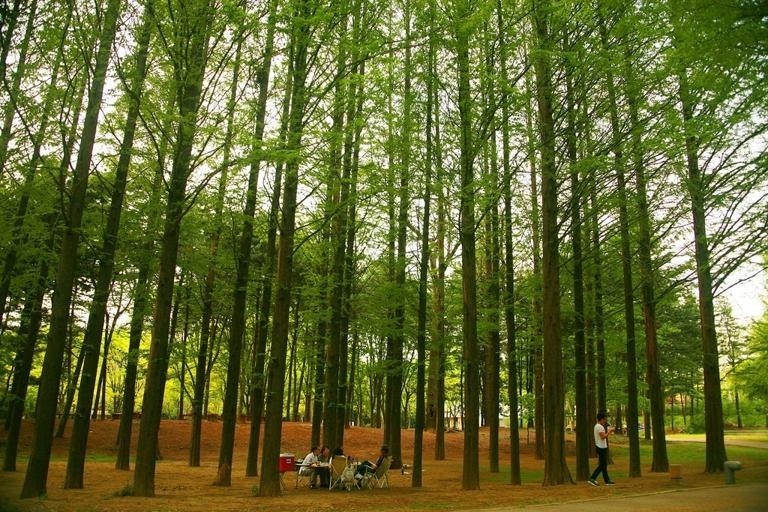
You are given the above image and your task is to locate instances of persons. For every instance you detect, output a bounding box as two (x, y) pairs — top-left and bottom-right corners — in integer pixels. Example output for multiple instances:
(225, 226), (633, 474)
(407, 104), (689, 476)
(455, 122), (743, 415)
(298, 446), (322, 489)
(316, 445), (330, 487)
(335, 448), (346, 490)
(354, 444), (389, 480)
(587, 412), (616, 487)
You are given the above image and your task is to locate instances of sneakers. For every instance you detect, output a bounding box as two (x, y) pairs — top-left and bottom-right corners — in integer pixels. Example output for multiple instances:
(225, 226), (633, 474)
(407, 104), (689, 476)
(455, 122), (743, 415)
(604, 480), (615, 485)
(588, 477), (601, 487)
(309, 484), (317, 489)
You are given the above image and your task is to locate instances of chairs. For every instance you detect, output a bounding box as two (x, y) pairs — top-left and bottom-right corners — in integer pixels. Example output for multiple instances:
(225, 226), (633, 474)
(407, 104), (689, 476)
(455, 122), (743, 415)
(296, 455), (393, 492)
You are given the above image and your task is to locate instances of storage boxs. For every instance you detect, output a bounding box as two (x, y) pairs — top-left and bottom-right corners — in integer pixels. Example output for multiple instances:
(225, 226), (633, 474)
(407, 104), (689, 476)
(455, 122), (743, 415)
(279, 453), (295, 472)
